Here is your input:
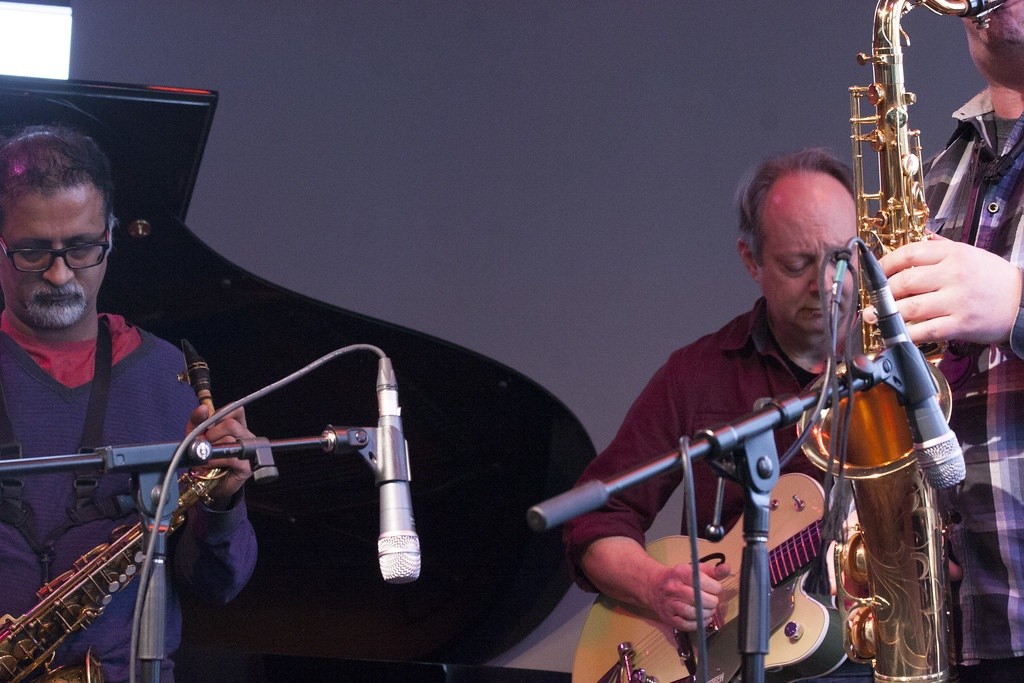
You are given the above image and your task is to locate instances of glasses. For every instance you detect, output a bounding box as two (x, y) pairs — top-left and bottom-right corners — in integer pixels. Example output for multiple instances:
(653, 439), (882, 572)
(0, 225), (109, 272)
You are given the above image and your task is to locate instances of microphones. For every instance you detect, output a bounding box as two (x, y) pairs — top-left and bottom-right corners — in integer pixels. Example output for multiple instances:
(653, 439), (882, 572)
(378, 357), (421, 584)
(859, 251), (966, 489)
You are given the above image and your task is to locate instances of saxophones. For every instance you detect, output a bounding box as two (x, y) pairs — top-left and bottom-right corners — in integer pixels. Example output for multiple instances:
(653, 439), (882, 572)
(795, 0), (993, 682)
(0, 338), (226, 683)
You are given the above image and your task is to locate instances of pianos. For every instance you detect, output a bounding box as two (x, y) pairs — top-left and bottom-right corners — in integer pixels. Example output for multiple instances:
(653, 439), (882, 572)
(0, 75), (596, 683)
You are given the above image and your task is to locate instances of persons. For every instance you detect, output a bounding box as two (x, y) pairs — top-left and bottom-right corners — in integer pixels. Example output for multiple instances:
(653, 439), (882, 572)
(863, 1), (1023, 682)
(0, 126), (258, 683)
(563, 150), (959, 683)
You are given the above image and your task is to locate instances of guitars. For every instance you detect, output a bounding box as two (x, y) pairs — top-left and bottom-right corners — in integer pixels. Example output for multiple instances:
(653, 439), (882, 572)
(571, 471), (850, 683)
